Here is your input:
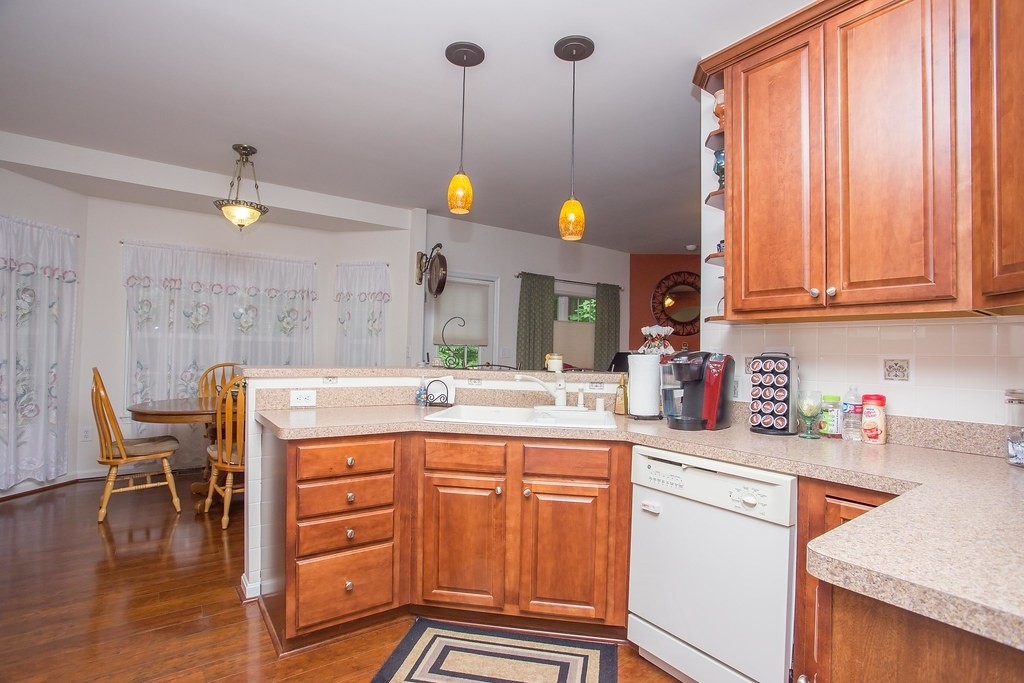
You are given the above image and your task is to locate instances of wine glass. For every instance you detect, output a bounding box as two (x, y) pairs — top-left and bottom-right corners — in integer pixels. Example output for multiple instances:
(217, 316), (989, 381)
(798, 390), (822, 439)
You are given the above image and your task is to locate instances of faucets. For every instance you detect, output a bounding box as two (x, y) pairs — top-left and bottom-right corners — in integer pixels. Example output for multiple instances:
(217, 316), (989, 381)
(515, 373), (567, 406)
(577, 386), (585, 407)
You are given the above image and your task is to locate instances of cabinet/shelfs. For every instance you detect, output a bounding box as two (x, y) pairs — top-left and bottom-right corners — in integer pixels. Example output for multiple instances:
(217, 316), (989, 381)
(692, 0), (1023, 321)
(410, 434), (632, 643)
(794, 474), (1024, 683)
(261, 432), (407, 655)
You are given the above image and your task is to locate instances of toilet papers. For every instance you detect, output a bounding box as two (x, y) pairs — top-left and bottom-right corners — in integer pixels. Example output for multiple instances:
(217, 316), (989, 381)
(627, 353), (661, 416)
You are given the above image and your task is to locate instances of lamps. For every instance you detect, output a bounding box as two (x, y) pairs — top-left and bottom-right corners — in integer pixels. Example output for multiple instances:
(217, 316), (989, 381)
(416, 242), (447, 298)
(444, 42), (484, 213)
(554, 34), (595, 242)
(213, 144), (270, 232)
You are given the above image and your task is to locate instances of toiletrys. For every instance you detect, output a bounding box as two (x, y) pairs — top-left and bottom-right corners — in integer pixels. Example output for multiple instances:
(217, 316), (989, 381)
(614, 372), (628, 415)
(415, 374), (427, 406)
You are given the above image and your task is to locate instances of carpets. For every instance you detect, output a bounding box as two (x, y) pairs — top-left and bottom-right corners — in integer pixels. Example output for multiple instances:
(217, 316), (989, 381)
(372, 619), (618, 683)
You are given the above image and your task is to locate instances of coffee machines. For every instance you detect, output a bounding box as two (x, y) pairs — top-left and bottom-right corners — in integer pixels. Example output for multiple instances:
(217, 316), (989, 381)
(659, 350), (735, 430)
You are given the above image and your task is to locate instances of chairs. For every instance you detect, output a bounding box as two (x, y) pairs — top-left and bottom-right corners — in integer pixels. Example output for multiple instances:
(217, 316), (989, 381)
(204, 375), (247, 529)
(92, 367), (181, 524)
(197, 362), (249, 481)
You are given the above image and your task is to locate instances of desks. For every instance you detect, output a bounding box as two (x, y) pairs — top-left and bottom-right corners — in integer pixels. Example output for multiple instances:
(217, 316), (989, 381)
(126, 395), (251, 495)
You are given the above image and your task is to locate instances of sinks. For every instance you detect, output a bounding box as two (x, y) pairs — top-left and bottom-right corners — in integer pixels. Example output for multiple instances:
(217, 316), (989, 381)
(423, 403), (618, 428)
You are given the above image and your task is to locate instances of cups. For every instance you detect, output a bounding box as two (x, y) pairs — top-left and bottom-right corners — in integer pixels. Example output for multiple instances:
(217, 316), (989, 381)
(547, 352), (563, 373)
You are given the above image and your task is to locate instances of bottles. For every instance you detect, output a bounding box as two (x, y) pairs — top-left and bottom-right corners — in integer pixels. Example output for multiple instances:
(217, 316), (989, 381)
(1004, 388), (1024, 468)
(842, 385), (863, 442)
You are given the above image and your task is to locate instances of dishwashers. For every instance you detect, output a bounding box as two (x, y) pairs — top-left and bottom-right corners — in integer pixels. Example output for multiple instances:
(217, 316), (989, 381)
(626, 444), (799, 683)
